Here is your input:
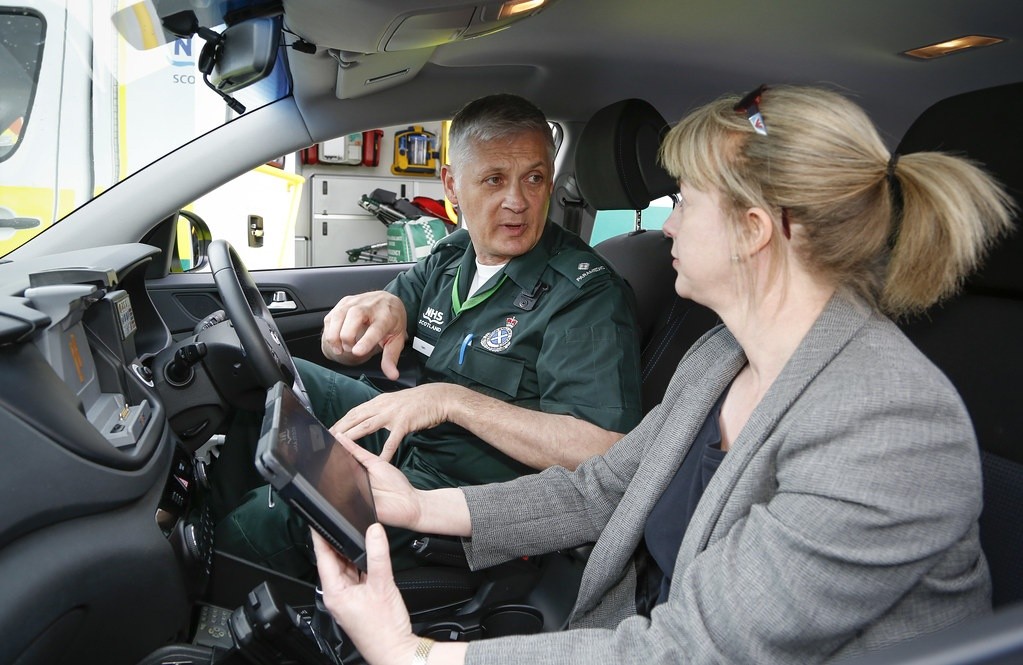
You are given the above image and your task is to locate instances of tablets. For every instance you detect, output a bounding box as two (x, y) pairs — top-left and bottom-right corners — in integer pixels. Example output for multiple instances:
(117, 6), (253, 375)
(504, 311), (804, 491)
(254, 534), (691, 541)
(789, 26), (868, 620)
(256, 381), (379, 574)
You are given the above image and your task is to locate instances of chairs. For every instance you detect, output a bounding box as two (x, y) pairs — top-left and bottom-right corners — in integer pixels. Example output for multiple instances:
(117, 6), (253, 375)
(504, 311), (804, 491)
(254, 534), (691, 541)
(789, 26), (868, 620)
(575, 98), (702, 380)
(883, 81), (1021, 606)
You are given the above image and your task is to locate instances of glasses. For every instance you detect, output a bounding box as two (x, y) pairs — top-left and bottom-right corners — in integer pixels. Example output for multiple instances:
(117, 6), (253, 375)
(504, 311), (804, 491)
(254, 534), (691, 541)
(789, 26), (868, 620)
(733, 82), (791, 240)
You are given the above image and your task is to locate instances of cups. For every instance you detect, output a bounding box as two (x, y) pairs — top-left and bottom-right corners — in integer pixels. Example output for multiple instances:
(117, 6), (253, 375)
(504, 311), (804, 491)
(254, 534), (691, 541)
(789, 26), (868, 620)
(408, 136), (428, 165)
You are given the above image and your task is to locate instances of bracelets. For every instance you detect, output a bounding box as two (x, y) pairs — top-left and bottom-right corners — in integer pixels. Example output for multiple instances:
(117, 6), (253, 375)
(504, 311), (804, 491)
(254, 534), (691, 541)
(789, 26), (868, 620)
(414, 636), (434, 665)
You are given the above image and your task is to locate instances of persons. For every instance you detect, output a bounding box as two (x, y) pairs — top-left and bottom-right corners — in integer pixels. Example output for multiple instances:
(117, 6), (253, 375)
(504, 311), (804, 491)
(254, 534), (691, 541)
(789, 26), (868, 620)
(210, 93), (644, 608)
(307, 81), (1018, 665)
